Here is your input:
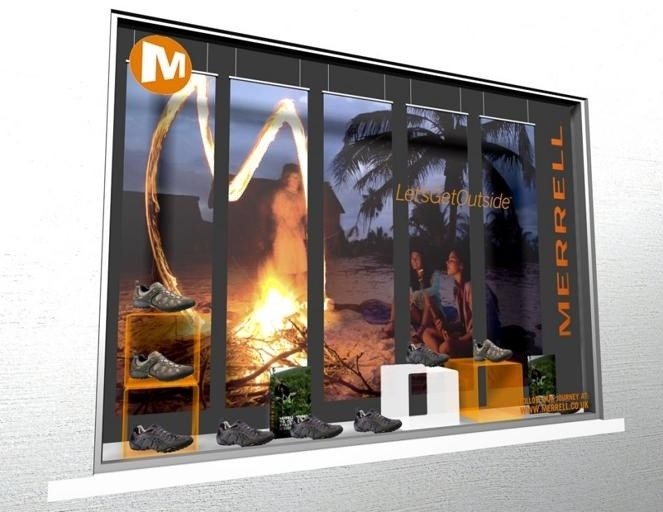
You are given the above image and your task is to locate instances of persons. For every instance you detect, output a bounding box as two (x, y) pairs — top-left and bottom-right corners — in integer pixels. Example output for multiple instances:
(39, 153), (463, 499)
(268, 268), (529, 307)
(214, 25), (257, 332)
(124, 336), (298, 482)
(420, 245), (499, 357)
(271, 163), (307, 279)
(383, 245), (447, 346)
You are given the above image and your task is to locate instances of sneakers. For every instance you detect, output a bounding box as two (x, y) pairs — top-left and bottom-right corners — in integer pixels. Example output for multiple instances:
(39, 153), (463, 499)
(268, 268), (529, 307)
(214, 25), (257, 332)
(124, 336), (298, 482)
(354, 407), (402, 433)
(405, 343), (449, 367)
(130, 350), (194, 381)
(216, 419), (275, 447)
(289, 415), (343, 440)
(473, 339), (513, 362)
(129, 423), (193, 453)
(132, 280), (196, 312)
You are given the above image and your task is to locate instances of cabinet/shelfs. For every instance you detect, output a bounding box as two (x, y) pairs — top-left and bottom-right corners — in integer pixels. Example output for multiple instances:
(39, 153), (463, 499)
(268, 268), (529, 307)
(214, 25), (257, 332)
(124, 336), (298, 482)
(119, 309), (200, 458)
